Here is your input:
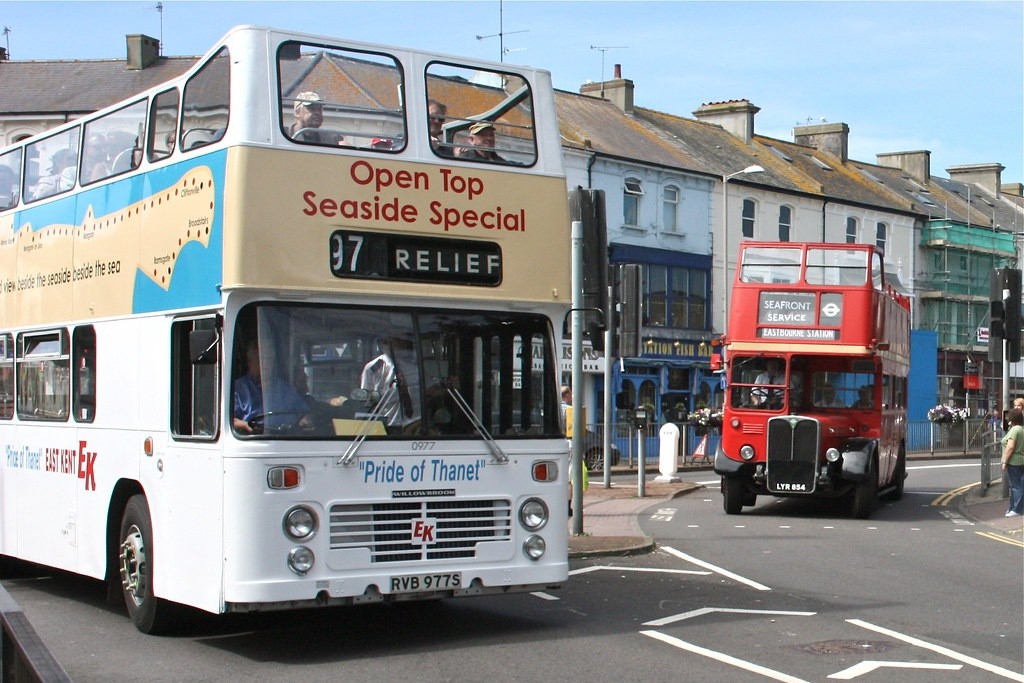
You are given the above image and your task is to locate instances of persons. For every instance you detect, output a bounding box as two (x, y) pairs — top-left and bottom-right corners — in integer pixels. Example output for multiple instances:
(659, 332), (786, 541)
(294, 368), (316, 406)
(462, 122), (523, 164)
(751, 357), (795, 407)
(1001, 408), (1024, 517)
(849, 387), (874, 408)
(816, 383), (843, 407)
(361, 329), (460, 431)
(231, 338), (314, 434)
(989, 408), (1002, 454)
(428, 98), (456, 156)
(0, 128), (186, 214)
(283, 91), (344, 145)
(560, 385), (573, 517)
(370, 137), (393, 150)
(1013, 397), (1024, 415)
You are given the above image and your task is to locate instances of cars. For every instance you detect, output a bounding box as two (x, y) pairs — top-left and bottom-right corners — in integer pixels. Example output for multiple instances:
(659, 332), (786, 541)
(583, 428), (621, 471)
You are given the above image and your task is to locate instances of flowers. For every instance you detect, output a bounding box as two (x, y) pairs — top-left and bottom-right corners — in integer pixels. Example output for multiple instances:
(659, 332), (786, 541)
(688, 406), (722, 436)
(928, 404), (967, 428)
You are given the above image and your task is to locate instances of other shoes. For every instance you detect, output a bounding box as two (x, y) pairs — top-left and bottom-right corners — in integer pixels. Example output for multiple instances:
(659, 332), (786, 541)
(1005, 510), (1019, 517)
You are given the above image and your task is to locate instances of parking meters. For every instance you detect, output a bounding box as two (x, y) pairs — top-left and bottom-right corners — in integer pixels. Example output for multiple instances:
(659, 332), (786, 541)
(634, 406), (649, 497)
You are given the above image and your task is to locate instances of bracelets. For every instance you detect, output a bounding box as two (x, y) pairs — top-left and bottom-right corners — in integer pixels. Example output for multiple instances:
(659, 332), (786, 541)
(1001, 461), (1007, 464)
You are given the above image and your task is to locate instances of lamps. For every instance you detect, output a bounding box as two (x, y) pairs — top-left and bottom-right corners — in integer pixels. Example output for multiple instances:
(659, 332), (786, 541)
(648, 334), (654, 346)
(699, 336), (707, 348)
(673, 336), (680, 347)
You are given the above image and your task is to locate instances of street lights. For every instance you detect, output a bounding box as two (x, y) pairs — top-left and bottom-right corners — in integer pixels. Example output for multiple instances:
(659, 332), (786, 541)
(722, 165), (764, 417)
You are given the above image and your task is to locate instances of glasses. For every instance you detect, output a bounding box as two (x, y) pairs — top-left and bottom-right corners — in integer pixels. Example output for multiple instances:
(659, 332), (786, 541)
(87, 144), (105, 154)
(822, 390), (834, 395)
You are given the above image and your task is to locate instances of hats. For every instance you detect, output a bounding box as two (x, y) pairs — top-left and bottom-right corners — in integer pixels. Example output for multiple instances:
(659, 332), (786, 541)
(428, 114), (445, 122)
(469, 122), (496, 134)
(373, 137), (393, 148)
(294, 92), (322, 108)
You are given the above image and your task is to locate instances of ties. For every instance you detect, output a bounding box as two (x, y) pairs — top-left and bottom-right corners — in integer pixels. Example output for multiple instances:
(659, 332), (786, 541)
(394, 362), (413, 419)
(768, 378), (774, 396)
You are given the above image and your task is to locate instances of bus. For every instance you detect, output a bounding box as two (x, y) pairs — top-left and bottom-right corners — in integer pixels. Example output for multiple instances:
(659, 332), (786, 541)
(0, 23), (610, 635)
(710, 240), (910, 519)
